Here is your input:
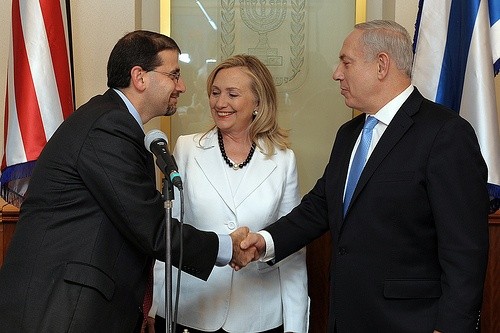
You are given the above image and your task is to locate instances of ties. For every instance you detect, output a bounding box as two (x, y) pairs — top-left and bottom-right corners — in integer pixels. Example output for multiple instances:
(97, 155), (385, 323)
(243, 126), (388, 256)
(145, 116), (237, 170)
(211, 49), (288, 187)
(344, 116), (379, 218)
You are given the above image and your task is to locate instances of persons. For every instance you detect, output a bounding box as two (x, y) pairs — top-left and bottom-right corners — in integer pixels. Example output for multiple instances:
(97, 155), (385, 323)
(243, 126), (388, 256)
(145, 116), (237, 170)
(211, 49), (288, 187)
(229, 20), (488, 333)
(0, 29), (259, 333)
(149, 54), (309, 333)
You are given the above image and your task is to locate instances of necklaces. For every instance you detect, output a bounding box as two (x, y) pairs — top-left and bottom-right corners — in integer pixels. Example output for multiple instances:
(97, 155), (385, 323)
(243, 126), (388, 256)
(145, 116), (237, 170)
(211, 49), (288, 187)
(218, 127), (255, 170)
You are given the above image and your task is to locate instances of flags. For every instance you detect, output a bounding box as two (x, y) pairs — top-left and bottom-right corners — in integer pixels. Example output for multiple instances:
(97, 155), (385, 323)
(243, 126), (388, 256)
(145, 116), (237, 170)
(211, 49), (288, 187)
(0, 0), (75, 210)
(410, 0), (500, 217)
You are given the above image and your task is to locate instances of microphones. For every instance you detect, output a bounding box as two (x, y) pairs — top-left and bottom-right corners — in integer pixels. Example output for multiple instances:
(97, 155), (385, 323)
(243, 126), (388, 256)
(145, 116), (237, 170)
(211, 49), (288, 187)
(144, 129), (183, 191)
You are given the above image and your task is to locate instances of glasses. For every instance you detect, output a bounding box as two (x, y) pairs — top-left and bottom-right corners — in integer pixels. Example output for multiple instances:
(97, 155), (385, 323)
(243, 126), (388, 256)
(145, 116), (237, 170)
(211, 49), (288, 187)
(149, 69), (180, 83)
